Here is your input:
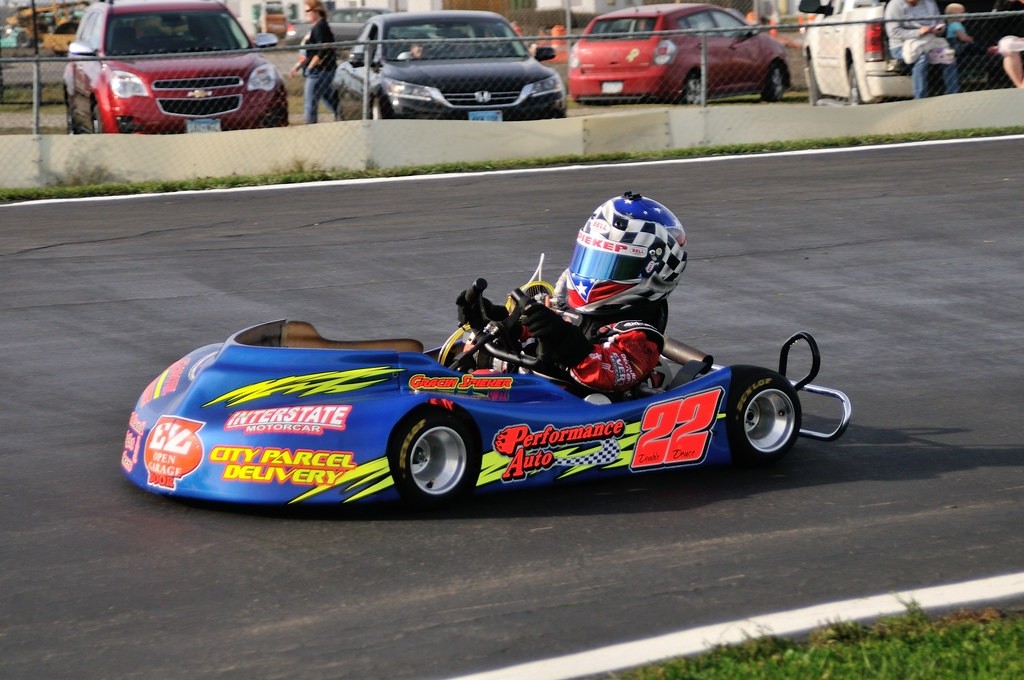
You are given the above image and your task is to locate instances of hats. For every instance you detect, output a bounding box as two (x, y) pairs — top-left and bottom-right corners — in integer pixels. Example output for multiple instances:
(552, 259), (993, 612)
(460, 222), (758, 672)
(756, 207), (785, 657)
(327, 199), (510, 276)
(307, 4), (325, 12)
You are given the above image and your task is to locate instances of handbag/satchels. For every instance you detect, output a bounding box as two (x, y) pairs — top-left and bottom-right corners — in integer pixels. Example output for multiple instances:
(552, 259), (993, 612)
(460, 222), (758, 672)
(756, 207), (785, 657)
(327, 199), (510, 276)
(901, 33), (951, 64)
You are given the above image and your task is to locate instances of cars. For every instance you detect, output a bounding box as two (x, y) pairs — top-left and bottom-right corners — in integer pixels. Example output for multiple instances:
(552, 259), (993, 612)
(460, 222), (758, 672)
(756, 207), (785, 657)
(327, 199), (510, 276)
(798, 0), (989, 106)
(286, 7), (393, 47)
(567, 3), (792, 105)
(333, 9), (567, 121)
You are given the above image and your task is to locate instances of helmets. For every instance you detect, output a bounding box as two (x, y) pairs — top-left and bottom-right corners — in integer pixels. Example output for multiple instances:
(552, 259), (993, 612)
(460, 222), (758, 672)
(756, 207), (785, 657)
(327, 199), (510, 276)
(566, 191), (687, 314)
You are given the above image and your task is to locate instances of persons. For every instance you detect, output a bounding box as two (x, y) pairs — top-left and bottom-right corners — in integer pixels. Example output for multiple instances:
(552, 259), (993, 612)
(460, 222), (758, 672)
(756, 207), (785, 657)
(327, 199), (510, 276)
(454, 190), (688, 400)
(883, 0), (1024, 99)
(288, 0), (345, 125)
(396, 42), (425, 61)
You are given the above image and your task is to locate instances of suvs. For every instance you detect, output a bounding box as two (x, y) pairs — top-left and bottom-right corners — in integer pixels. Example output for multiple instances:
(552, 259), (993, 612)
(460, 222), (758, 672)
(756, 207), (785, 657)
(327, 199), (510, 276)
(62, 1), (288, 136)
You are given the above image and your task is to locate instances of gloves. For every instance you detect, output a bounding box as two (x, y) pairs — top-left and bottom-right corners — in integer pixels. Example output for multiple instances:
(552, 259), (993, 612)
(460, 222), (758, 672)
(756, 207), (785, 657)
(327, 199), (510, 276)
(456, 290), (509, 328)
(522, 303), (594, 372)
